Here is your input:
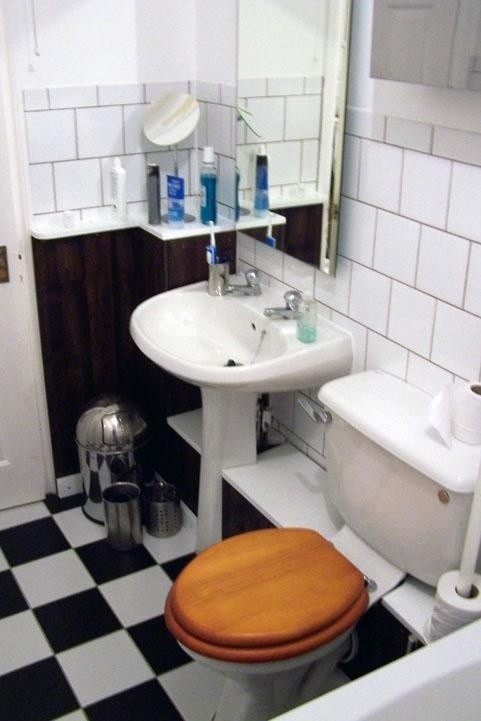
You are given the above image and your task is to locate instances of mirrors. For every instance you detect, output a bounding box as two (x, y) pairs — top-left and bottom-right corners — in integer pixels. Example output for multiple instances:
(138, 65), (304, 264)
(235, 0), (353, 277)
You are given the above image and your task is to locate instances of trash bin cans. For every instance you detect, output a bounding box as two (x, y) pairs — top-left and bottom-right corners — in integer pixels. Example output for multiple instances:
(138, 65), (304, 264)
(75, 402), (148, 526)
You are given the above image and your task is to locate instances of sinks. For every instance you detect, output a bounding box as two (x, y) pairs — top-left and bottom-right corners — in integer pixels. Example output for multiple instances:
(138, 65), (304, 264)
(130, 275), (352, 389)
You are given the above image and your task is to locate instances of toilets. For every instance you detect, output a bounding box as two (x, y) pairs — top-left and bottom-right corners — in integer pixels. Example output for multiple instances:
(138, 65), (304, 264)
(165, 374), (480, 721)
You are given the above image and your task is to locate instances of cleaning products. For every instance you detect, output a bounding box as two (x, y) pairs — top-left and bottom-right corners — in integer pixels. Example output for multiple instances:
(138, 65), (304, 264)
(109, 159), (128, 221)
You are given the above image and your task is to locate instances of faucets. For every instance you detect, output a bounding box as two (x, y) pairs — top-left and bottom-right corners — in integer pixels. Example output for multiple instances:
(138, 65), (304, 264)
(264, 291), (305, 322)
(227, 270), (263, 298)
